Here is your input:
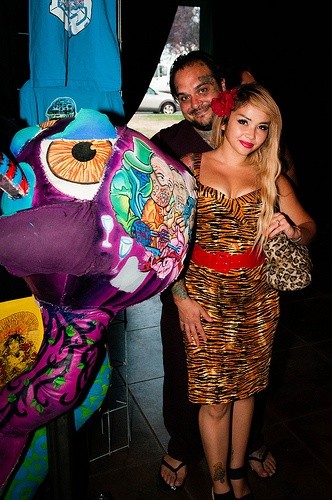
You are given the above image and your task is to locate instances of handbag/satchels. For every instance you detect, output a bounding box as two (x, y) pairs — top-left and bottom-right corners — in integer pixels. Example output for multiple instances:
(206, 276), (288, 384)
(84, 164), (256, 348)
(261, 180), (314, 292)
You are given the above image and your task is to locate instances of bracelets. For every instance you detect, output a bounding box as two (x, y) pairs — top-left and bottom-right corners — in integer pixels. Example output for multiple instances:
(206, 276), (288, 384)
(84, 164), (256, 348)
(290, 225), (302, 242)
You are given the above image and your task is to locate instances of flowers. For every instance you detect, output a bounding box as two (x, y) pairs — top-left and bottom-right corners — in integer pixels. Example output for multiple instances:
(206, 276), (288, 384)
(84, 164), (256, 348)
(210, 89), (238, 118)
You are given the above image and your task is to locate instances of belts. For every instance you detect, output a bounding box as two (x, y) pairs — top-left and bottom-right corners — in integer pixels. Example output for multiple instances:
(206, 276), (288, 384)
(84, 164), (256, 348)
(192, 244), (265, 273)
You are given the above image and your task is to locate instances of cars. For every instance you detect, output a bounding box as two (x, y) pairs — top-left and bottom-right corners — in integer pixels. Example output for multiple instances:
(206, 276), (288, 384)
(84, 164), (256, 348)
(137, 87), (181, 115)
(149, 63), (172, 92)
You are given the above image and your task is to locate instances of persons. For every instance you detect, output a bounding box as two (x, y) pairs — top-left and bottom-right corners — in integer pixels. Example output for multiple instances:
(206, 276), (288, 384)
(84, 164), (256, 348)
(144, 51), (278, 489)
(170, 84), (316, 500)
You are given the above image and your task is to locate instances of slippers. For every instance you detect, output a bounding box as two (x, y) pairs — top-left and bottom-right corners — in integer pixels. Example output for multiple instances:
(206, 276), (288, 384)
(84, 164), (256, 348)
(158, 453), (188, 493)
(250, 445), (280, 480)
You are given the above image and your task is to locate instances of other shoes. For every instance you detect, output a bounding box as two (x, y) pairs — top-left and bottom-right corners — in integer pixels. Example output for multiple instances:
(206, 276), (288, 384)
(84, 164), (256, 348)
(210, 466), (254, 500)
